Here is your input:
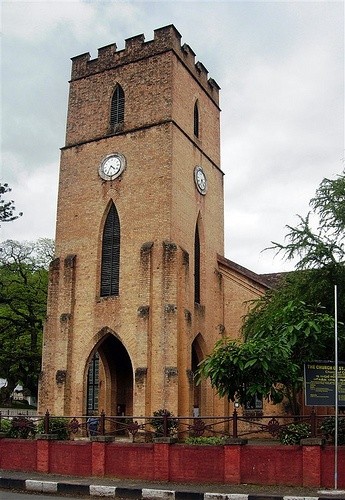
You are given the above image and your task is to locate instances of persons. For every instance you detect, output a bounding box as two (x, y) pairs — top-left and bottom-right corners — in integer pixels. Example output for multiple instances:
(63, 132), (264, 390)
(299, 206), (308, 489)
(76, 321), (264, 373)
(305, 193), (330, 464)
(87, 414), (99, 436)
(122, 403), (134, 415)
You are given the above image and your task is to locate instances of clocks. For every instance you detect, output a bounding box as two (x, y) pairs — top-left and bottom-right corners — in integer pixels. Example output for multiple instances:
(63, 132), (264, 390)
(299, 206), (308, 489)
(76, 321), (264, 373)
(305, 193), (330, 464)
(98, 152), (126, 181)
(193, 164), (209, 195)
